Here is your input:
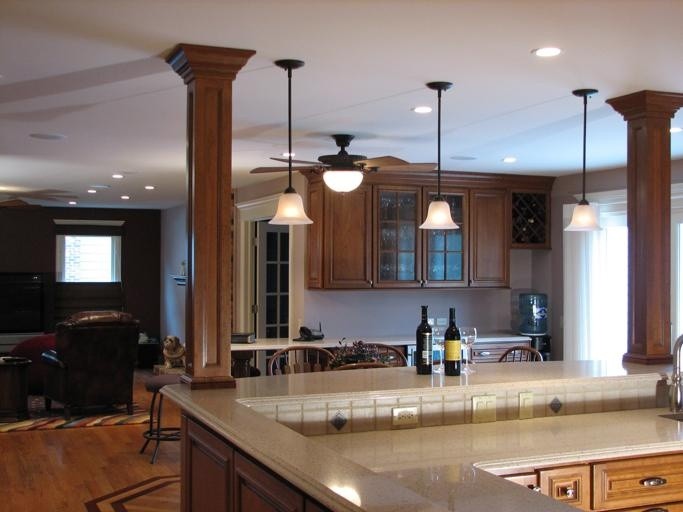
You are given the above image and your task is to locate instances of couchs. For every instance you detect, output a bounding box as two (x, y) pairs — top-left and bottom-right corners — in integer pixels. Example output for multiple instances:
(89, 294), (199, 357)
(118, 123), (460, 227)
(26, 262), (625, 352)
(12, 333), (57, 397)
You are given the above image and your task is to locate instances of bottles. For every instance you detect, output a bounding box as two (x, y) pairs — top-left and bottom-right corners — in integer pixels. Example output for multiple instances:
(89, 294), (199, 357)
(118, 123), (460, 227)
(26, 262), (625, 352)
(417, 305), (433, 376)
(444, 308), (460, 378)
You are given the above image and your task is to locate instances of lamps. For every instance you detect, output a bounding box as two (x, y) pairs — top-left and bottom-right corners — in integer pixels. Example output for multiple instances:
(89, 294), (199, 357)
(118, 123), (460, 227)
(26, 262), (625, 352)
(418, 82), (461, 229)
(564, 89), (604, 233)
(318, 164), (366, 193)
(268, 59), (314, 225)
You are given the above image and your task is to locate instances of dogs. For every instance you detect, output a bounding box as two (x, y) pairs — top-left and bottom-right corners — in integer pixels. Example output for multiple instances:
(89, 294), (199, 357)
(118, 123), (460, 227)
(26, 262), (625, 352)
(161, 335), (188, 370)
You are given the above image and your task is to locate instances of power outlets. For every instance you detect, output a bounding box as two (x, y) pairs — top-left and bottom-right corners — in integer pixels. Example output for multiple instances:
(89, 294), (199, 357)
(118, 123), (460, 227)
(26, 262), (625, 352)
(393, 407), (418, 426)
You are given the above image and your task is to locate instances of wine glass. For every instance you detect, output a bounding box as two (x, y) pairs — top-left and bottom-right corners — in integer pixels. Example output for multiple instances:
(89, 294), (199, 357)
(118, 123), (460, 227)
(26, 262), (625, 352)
(433, 328), (447, 375)
(460, 328), (477, 376)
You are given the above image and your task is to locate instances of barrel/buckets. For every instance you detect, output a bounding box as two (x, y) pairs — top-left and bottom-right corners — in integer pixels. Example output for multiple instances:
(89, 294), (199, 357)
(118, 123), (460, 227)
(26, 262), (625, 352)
(519, 294), (547, 333)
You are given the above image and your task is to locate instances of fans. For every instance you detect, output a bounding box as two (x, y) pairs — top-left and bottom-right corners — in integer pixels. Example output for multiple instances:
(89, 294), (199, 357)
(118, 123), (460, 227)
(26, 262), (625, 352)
(250, 154), (438, 178)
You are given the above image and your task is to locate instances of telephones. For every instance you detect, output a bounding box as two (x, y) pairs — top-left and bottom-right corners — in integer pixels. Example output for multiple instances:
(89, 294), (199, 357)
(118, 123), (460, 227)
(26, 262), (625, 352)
(298, 322), (324, 341)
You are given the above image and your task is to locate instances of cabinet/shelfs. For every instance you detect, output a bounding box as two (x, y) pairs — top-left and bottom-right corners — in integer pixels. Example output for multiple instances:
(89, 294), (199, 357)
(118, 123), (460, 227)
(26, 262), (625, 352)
(373, 170), (469, 289)
(306, 345), (408, 371)
(304, 182), (373, 289)
(468, 339), (532, 364)
(501, 452), (683, 512)
(470, 173), (512, 289)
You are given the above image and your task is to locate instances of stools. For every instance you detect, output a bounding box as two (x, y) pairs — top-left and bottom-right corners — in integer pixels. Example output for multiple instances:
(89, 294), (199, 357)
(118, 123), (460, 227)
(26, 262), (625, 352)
(140, 374), (182, 464)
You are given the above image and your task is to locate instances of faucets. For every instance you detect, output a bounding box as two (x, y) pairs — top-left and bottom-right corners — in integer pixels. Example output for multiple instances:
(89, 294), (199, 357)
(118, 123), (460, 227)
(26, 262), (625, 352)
(669, 335), (683, 418)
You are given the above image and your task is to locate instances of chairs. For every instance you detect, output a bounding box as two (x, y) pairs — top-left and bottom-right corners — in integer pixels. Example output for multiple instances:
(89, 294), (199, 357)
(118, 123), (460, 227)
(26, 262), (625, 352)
(334, 363), (390, 370)
(268, 346), (335, 377)
(40, 310), (140, 420)
(499, 346), (543, 362)
(354, 343), (408, 367)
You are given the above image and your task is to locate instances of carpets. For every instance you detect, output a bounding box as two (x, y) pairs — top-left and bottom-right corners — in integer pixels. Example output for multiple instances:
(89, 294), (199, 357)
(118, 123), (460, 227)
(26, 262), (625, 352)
(0, 395), (159, 432)
